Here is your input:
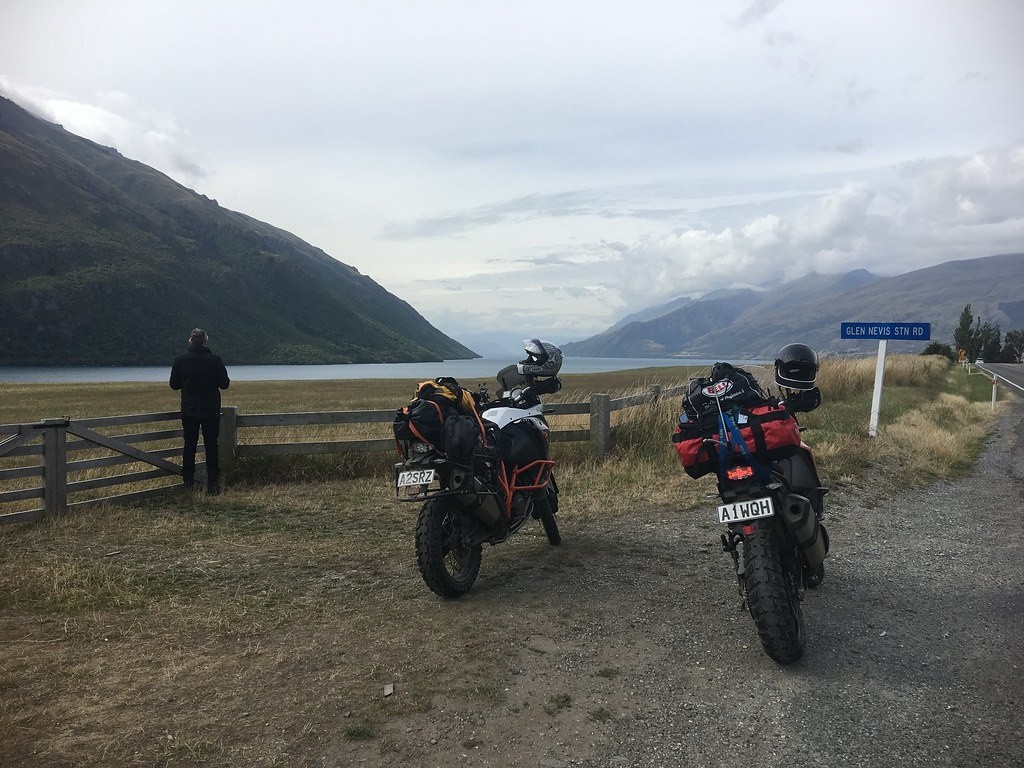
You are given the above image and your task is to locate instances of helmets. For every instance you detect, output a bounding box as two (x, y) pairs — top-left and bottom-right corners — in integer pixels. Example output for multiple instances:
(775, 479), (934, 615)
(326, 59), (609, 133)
(774, 343), (820, 391)
(517, 337), (564, 376)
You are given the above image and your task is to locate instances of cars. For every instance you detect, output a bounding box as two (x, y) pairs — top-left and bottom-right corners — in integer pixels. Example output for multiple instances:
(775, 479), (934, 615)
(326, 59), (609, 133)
(974, 357), (984, 364)
(958, 356), (970, 364)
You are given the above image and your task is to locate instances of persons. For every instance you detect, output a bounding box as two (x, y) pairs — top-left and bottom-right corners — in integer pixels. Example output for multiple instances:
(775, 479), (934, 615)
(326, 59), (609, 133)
(169, 327), (229, 498)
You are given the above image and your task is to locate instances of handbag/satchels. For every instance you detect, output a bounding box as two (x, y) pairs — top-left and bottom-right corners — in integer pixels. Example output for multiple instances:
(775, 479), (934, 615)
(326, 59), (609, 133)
(671, 364), (802, 480)
(393, 377), (479, 460)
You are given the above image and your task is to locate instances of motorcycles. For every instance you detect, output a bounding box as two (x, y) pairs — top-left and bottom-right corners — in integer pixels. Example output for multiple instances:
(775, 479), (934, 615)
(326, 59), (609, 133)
(393, 362), (563, 599)
(677, 362), (832, 667)
